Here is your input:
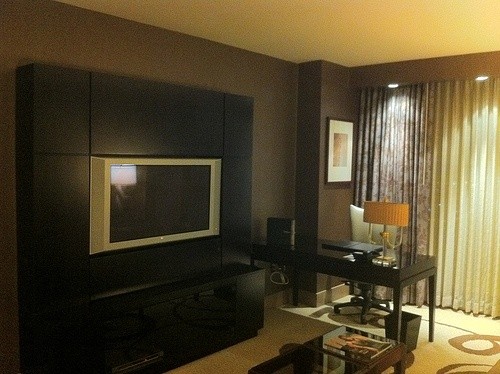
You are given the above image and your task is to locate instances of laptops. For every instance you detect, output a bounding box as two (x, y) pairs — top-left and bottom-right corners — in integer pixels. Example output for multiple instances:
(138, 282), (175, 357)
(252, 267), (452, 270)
(322, 239), (383, 254)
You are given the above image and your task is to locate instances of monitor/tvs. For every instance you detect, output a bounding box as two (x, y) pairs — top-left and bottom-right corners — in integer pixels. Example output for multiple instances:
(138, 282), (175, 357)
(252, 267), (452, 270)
(90, 156), (221, 255)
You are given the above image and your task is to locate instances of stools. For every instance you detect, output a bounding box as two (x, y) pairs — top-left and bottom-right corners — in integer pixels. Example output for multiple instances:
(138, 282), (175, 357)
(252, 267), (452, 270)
(334, 205), (396, 325)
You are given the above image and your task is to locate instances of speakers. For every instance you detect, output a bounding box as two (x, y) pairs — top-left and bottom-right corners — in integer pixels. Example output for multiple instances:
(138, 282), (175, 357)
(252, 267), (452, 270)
(267, 216), (296, 246)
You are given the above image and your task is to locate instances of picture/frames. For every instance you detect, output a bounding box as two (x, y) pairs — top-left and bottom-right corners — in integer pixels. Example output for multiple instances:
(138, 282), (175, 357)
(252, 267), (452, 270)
(326, 116), (354, 184)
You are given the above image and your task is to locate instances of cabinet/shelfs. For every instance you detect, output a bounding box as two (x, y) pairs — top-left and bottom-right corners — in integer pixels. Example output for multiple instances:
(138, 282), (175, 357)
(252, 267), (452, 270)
(19, 261), (264, 374)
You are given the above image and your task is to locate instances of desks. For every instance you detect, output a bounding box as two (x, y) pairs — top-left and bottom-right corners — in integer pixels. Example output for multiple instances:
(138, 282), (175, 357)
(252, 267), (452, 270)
(250, 233), (436, 346)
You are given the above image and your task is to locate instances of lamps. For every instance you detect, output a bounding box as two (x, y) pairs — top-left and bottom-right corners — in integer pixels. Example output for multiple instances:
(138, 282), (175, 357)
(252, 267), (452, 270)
(364, 201), (408, 261)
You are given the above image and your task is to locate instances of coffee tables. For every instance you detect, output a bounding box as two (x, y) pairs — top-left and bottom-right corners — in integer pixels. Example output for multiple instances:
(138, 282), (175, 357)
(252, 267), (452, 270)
(249, 328), (414, 374)
